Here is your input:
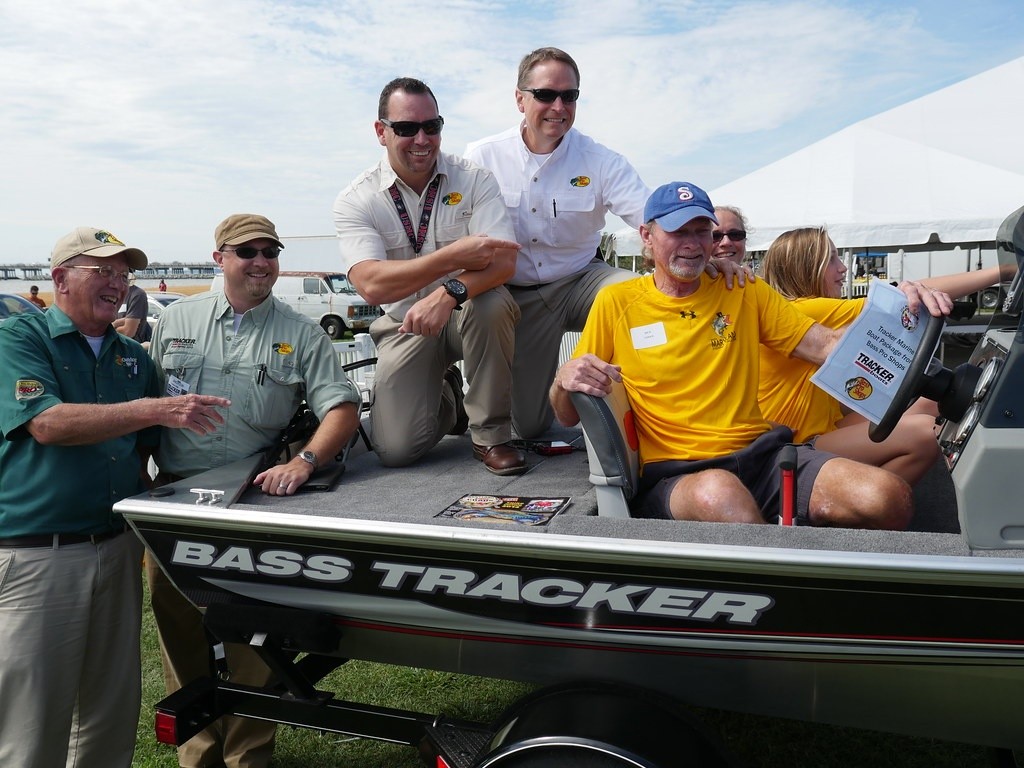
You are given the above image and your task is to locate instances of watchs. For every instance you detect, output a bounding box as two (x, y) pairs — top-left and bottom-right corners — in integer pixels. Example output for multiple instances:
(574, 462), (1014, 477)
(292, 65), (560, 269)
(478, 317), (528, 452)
(298, 450), (319, 469)
(441, 278), (468, 311)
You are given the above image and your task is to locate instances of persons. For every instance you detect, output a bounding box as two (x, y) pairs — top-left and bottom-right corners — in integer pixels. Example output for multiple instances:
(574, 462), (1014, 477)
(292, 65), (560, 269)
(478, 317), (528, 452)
(463, 43), (758, 446)
(545, 178), (957, 531)
(708, 205), (750, 264)
(0, 226), (234, 768)
(110, 267), (152, 344)
(147, 212), (361, 768)
(330, 77), (532, 476)
(158, 279), (166, 291)
(753, 225), (1019, 484)
(17, 285), (47, 312)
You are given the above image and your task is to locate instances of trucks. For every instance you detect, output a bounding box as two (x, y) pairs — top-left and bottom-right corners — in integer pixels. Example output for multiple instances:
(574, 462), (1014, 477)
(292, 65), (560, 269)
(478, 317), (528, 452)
(885, 246), (1012, 310)
(210, 269), (383, 341)
(109, 203), (1024, 767)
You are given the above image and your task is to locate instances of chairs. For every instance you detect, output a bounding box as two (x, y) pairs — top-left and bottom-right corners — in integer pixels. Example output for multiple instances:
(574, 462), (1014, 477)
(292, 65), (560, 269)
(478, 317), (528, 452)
(569, 374), (640, 521)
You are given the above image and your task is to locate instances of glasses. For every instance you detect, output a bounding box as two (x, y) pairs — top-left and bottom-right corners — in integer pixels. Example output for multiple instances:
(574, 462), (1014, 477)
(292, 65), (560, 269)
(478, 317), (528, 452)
(31, 292), (39, 295)
(222, 246), (282, 258)
(522, 88), (580, 103)
(66, 264), (133, 285)
(712, 231), (748, 244)
(380, 115), (444, 137)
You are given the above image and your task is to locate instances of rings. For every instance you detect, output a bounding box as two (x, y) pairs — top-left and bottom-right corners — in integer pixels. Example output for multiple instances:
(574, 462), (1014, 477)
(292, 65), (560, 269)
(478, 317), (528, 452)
(279, 484), (287, 488)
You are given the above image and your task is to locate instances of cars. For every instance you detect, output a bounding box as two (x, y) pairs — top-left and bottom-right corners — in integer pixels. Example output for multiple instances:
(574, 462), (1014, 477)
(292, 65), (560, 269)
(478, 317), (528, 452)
(0, 291), (47, 322)
(117, 290), (189, 332)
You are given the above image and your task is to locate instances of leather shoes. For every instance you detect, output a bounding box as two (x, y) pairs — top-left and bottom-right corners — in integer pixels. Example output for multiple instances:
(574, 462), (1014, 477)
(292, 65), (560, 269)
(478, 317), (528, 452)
(472, 443), (528, 473)
(443, 364), (467, 436)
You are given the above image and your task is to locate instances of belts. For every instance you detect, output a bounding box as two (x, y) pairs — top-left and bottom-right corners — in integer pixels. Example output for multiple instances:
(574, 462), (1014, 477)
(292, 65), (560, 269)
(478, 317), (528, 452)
(0, 520), (130, 549)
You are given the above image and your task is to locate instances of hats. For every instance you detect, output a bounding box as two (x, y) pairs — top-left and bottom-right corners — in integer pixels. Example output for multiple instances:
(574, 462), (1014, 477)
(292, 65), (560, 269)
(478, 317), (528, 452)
(216, 214), (284, 249)
(52, 227), (149, 271)
(645, 180), (719, 232)
